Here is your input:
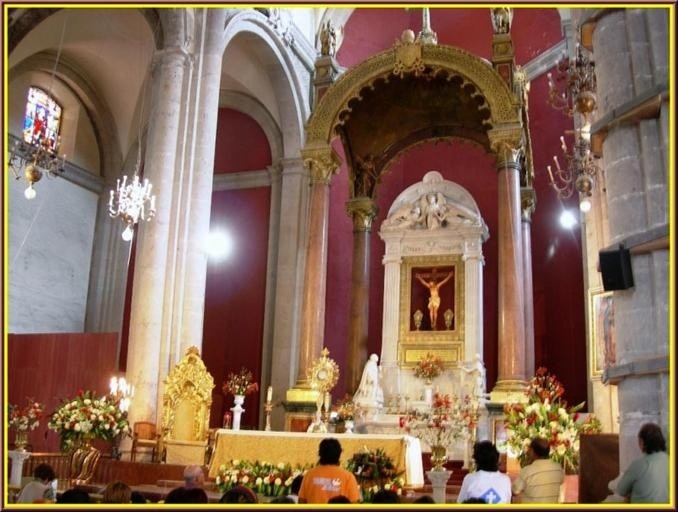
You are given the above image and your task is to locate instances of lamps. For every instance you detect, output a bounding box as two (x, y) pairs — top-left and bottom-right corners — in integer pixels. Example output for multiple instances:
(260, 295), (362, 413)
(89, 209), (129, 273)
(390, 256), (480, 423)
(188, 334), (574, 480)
(130, 422), (161, 463)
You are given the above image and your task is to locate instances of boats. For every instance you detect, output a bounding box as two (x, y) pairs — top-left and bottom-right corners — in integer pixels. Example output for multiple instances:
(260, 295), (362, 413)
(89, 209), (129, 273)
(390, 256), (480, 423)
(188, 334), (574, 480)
(599, 246), (635, 291)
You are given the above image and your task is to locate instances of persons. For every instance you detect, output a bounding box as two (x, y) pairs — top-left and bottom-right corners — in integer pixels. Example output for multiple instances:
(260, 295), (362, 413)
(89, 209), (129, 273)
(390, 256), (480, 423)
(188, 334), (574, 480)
(352, 351), (385, 408)
(165, 486), (207, 503)
(103, 479), (131, 504)
(380, 204), (424, 232)
(219, 487), (258, 502)
(511, 437), (568, 505)
(58, 488), (89, 503)
(419, 191), (448, 231)
(455, 440), (512, 504)
(373, 488), (400, 504)
(327, 495), (351, 504)
(182, 463), (205, 490)
(286, 474), (305, 503)
(298, 438), (362, 503)
(270, 498), (295, 503)
(615, 423), (670, 503)
(461, 497), (486, 504)
(458, 354), (487, 403)
(131, 487), (163, 504)
(414, 495), (435, 503)
(432, 202), (479, 225)
(15, 462), (56, 503)
(416, 271), (456, 329)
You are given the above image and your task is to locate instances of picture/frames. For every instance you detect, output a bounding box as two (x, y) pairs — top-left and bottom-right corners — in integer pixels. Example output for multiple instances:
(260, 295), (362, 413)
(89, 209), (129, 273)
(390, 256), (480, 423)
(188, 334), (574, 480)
(207, 428), (424, 498)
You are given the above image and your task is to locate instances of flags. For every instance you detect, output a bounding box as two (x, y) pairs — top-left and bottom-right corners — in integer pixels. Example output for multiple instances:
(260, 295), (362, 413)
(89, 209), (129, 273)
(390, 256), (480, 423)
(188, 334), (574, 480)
(424, 378), (431, 385)
(14, 430), (28, 452)
(77, 431), (95, 448)
(232, 395), (245, 410)
(429, 446), (449, 472)
(344, 420), (354, 433)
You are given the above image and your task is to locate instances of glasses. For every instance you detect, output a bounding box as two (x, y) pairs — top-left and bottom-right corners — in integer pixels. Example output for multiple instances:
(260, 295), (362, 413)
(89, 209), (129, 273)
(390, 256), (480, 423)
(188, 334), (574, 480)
(545, 113), (597, 199)
(544, 9), (597, 128)
(7, 8), (70, 200)
(108, 8), (162, 241)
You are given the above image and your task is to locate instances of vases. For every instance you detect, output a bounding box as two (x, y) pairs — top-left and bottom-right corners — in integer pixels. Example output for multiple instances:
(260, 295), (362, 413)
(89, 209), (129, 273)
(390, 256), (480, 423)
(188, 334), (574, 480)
(221, 366), (258, 396)
(495, 367), (605, 476)
(411, 352), (448, 381)
(8, 395), (46, 432)
(331, 393), (368, 425)
(42, 387), (133, 458)
(399, 391), (479, 450)
(344, 445), (415, 504)
(214, 458), (320, 498)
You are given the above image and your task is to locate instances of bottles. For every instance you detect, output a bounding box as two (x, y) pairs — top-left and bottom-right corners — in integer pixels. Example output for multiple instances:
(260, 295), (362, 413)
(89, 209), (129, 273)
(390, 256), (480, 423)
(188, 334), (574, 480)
(319, 438), (342, 453)
